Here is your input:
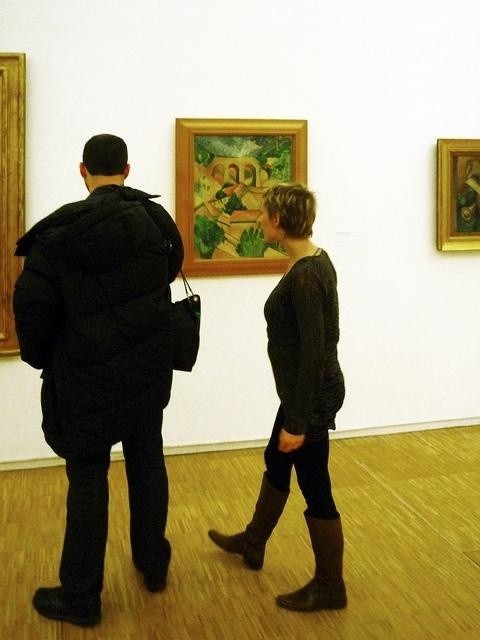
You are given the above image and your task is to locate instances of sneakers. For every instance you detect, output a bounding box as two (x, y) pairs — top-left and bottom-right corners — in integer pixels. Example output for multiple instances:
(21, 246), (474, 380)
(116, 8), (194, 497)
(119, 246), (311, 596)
(30, 584), (104, 629)
(137, 538), (171, 593)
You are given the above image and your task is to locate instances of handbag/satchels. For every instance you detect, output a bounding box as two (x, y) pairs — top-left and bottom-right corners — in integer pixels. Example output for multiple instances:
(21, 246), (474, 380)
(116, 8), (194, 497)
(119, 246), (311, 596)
(171, 293), (201, 372)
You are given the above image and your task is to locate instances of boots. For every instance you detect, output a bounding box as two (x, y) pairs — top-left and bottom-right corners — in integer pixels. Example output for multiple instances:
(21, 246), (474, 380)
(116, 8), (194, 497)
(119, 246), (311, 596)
(207, 473), (291, 570)
(273, 508), (348, 611)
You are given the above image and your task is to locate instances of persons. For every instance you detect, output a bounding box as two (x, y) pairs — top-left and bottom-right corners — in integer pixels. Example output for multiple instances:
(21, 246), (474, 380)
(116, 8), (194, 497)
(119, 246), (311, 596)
(209, 180), (351, 614)
(11, 130), (184, 629)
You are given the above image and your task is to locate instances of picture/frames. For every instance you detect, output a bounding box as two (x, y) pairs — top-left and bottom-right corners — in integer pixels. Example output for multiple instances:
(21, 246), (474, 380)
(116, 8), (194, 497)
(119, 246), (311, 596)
(176, 118), (308, 277)
(0, 52), (27, 356)
(436, 139), (480, 252)
